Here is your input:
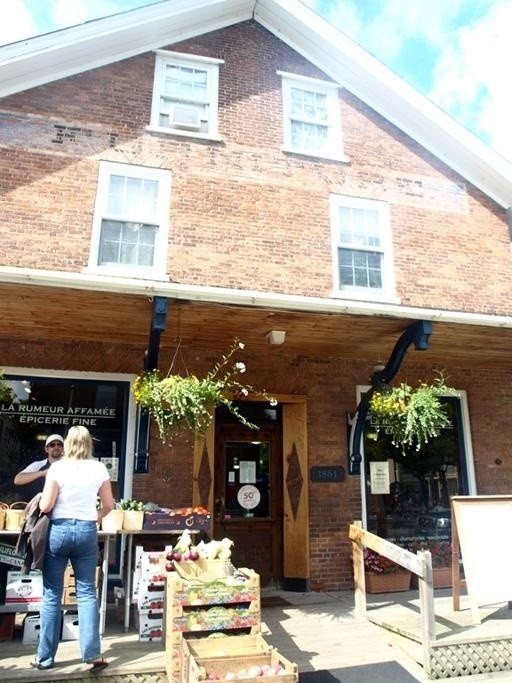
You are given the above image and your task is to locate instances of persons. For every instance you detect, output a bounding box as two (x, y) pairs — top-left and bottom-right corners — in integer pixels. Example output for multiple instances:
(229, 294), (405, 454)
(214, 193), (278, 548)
(28, 424), (115, 674)
(14, 433), (71, 574)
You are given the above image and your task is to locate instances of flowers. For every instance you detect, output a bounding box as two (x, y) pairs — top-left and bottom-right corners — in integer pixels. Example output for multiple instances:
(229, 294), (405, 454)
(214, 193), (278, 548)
(360, 534), (462, 573)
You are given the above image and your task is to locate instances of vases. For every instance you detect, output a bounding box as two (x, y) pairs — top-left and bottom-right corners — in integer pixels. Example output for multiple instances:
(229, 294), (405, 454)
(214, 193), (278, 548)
(362, 565), (460, 594)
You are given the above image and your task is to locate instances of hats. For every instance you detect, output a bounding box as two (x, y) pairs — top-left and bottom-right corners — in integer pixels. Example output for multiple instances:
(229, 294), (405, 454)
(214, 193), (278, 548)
(45, 433), (64, 446)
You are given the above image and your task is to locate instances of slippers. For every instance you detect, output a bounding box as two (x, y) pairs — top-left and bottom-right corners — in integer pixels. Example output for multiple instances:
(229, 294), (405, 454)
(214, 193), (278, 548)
(88, 657), (109, 674)
(28, 654), (49, 669)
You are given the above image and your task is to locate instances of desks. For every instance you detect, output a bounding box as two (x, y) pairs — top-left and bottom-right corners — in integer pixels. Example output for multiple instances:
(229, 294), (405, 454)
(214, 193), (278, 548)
(1, 528), (200, 644)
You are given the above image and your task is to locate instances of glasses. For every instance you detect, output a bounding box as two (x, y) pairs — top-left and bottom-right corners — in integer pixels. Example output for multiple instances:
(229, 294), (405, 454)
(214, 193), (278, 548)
(46, 442), (63, 448)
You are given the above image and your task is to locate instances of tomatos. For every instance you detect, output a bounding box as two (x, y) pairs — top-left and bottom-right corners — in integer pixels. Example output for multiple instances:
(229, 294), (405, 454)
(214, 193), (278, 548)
(170, 505), (209, 517)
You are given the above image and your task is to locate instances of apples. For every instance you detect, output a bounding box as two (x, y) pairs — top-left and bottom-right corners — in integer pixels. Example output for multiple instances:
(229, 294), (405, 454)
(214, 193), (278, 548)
(199, 662), (286, 681)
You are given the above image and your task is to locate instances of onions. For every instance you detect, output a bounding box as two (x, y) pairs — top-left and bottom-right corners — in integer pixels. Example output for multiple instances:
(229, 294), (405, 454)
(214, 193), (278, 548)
(166, 549), (200, 572)
